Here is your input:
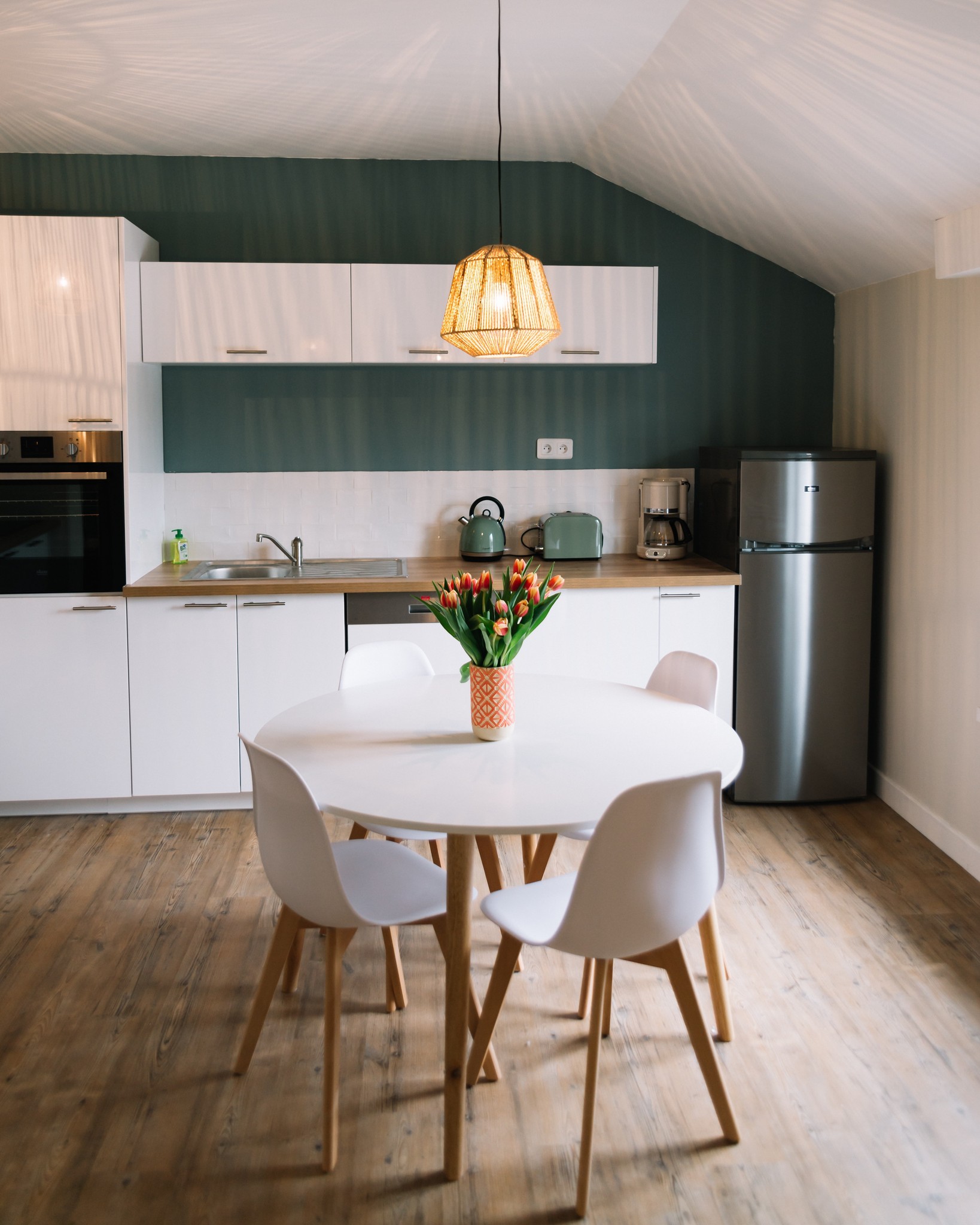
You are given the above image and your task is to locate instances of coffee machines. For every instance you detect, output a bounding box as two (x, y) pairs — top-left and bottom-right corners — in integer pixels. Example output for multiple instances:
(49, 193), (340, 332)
(637, 476), (690, 562)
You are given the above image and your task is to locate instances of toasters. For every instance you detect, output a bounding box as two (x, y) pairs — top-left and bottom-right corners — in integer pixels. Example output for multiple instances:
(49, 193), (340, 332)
(534, 511), (604, 561)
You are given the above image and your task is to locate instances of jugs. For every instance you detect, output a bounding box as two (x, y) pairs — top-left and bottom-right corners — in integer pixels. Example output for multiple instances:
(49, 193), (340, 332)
(643, 515), (693, 547)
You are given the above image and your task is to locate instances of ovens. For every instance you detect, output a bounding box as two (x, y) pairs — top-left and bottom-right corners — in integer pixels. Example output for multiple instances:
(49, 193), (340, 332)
(0, 429), (126, 595)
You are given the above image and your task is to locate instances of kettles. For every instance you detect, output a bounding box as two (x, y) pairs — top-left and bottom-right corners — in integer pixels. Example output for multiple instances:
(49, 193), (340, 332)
(456, 496), (507, 563)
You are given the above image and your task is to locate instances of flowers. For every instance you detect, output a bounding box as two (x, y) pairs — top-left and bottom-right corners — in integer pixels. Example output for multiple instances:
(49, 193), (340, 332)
(409, 555), (566, 685)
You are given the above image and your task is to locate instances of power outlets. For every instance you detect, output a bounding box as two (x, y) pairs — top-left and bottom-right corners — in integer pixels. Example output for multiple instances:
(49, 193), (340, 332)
(537, 439), (573, 459)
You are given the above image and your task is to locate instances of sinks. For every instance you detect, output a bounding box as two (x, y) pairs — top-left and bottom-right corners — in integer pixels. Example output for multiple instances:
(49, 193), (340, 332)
(198, 566), (290, 578)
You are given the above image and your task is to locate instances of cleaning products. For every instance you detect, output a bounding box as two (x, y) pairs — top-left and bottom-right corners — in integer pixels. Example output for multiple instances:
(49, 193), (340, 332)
(169, 529), (188, 564)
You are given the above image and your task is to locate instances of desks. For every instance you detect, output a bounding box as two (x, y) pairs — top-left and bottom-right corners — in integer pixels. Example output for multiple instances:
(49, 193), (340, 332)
(253, 679), (745, 1181)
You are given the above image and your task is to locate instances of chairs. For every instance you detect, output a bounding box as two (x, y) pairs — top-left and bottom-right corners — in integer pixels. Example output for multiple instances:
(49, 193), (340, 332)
(236, 641), (739, 1215)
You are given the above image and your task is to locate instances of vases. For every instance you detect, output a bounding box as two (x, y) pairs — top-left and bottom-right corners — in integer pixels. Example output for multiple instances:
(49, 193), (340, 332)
(468, 661), (515, 741)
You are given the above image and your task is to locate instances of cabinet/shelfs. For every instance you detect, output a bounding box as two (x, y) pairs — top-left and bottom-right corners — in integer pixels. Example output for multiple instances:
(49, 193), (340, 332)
(140, 261), (352, 365)
(0, 596), (131, 799)
(497, 587), (734, 728)
(499, 265), (659, 364)
(126, 592), (344, 796)
(350, 264), (504, 365)
(0, 215), (162, 435)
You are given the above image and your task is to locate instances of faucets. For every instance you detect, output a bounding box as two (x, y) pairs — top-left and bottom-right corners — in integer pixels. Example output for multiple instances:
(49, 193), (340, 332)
(256, 533), (303, 567)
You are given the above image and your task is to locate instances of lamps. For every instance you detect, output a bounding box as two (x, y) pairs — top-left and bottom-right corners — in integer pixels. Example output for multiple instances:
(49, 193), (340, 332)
(440, 0), (560, 358)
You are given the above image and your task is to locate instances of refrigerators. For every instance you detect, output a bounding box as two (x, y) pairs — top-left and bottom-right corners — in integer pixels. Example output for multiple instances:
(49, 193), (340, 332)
(695, 442), (879, 805)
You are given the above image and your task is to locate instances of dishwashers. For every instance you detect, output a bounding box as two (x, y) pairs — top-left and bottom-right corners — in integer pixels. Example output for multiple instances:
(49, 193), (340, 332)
(345, 591), (445, 623)
